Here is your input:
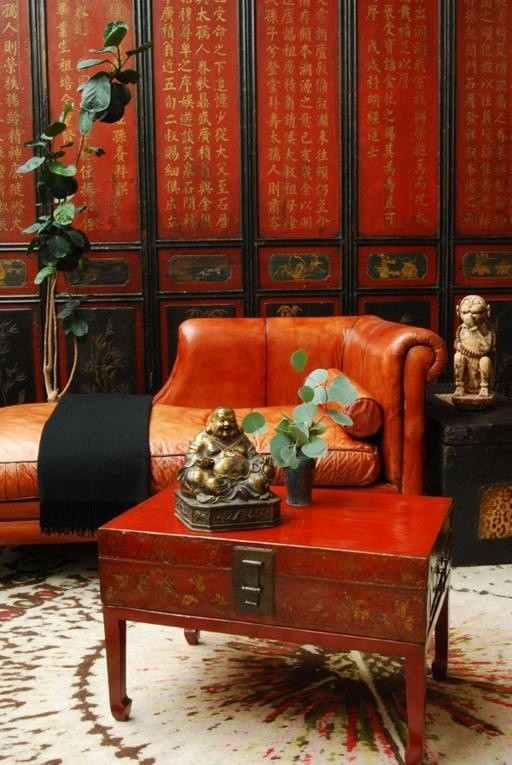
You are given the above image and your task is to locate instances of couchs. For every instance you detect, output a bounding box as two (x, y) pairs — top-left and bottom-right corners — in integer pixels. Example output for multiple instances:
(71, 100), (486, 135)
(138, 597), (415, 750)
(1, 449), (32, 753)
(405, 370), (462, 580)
(0, 314), (448, 546)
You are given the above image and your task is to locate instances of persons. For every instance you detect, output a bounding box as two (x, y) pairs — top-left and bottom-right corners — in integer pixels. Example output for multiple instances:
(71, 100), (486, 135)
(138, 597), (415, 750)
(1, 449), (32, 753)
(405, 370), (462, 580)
(454, 293), (501, 398)
(176, 406), (280, 503)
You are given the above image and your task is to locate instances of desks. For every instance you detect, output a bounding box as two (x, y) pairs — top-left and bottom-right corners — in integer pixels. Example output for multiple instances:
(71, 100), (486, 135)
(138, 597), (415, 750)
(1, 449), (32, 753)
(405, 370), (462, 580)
(97, 487), (455, 764)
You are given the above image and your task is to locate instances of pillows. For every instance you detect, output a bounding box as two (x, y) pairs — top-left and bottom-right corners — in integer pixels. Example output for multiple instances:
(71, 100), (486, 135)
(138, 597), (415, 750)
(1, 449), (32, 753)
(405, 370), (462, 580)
(317, 367), (384, 440)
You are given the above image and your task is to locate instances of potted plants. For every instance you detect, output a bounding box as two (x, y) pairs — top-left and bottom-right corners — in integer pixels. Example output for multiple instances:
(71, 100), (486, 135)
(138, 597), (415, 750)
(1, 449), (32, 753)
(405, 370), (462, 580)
(238, 350), (354, 508)
